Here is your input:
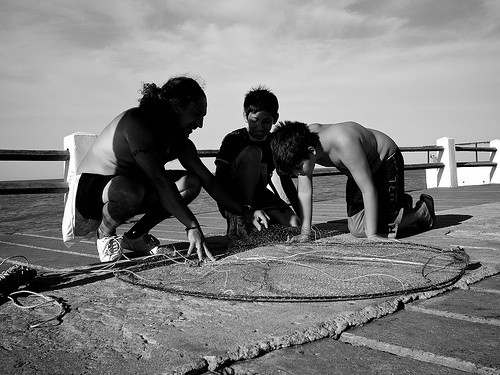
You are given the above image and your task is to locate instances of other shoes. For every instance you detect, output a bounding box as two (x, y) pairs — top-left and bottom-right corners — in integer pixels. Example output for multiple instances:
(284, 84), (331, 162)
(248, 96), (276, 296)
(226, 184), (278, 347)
(226, 213), (247, 240)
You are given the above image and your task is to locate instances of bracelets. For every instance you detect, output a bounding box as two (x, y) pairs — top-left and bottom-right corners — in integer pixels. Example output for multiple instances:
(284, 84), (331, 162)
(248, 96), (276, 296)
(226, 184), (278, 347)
(238, 204), (252, 216)
(185, 224), (200, 231)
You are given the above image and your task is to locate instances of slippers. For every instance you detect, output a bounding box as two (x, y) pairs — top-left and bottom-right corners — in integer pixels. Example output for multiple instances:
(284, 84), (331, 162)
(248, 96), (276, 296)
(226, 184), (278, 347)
(401, 193), (414, 213)
(419, 193), (436, 229)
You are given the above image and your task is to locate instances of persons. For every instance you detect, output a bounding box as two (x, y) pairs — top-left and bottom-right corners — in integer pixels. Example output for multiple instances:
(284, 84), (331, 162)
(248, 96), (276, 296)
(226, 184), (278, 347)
(270, 121), (436, 247)
(58, 77), (271, 267)
(213, 88), (301, 239)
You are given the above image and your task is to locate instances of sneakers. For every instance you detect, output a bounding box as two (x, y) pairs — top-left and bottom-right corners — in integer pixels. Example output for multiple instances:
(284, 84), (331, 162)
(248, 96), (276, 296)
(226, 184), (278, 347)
(122, 233), (172, 256)
(94, 230), (122, 263)
